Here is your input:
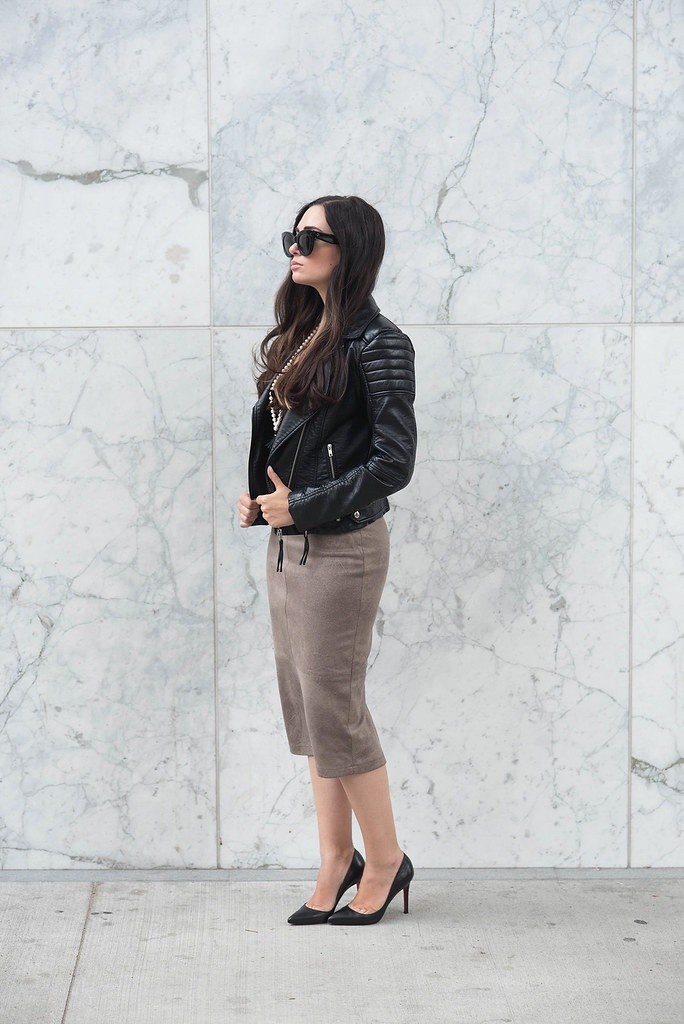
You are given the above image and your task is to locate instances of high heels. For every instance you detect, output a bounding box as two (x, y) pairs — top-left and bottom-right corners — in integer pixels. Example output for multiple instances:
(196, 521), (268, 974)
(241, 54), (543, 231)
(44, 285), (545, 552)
(287, 848), (365, 925)
(328, 853), (414, 925)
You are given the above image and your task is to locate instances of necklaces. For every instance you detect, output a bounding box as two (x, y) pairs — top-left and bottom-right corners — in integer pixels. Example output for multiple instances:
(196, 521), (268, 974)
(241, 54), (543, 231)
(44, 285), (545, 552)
(269, 323), (317, 432)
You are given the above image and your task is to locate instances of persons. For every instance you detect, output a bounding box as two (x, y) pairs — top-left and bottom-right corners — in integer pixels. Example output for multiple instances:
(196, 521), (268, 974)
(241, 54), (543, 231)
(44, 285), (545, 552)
(237, 196), (416, 926)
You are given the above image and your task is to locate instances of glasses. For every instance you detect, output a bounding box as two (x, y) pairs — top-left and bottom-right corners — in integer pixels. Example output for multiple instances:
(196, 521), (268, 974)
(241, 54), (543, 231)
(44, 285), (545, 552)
(281, 230), (338, 256)
